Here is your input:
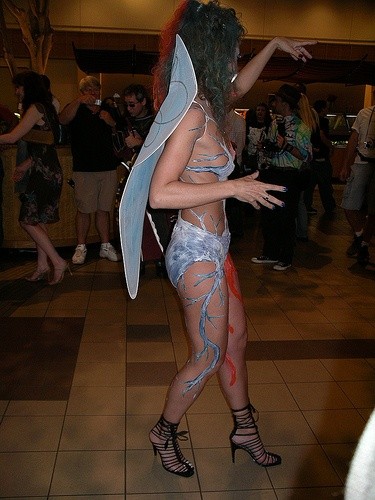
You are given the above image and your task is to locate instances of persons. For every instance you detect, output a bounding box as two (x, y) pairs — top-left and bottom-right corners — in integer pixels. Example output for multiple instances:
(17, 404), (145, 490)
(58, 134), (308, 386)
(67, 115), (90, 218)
(295, 93), (317, 241)
(11, 72), (60, 117)
(118, 0), (318, 478)
(295, 81), (319, 126)
(340, 90), (375, 267)
(304, 101), (336, 213)
(59, 76), (122, 264)
(251, 84), (313, 271)
(121, 83), (157, 154)
(241, 102), (271, 231)
(104, 97), (116, 108)
(0, 70), (72, 285)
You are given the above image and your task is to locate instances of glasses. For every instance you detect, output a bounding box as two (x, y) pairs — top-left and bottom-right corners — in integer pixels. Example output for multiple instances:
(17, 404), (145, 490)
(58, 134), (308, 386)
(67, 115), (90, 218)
(123, 98), (143, 108)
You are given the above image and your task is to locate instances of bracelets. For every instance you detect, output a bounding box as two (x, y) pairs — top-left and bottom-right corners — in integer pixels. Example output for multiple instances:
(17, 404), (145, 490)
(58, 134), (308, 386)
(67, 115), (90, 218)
(284, 144), (294, 152)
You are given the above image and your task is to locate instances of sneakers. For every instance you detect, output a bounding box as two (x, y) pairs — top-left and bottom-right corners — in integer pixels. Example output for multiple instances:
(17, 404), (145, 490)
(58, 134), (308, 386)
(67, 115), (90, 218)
(275, 261), (292, 270)
(72, 246), (88, 264)
(99, 245), (122, 261)
(251, 255), (279, 263)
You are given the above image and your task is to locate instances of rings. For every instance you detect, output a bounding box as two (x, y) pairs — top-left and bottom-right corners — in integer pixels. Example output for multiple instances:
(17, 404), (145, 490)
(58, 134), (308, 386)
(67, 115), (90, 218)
(299, 46), (302, 49)
(266, 194), (269, 200)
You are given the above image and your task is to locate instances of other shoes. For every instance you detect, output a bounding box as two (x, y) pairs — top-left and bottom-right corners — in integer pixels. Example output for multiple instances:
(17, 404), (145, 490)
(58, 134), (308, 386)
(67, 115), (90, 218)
(356, 245), (369, 263)
(346, 230), (367, 255)
(156, 260), (169, 280)
(139, 261), (147, 274)
(305, 206), (318, 213)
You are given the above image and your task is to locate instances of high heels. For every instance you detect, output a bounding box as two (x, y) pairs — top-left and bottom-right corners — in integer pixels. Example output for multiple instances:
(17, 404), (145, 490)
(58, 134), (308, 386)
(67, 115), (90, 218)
(149, 419), (195, 478)
(230, 404), (281, 465)
(49, 260), (73, 285)
(25, 264), (51, 283)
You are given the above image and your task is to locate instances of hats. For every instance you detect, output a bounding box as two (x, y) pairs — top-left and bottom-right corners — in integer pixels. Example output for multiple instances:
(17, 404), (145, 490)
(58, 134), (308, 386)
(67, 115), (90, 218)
(272, 84), (302, 110)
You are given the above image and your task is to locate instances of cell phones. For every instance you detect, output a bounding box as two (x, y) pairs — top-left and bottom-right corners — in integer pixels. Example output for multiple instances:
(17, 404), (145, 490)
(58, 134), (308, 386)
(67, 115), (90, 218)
(93, 98), (102, 106)
